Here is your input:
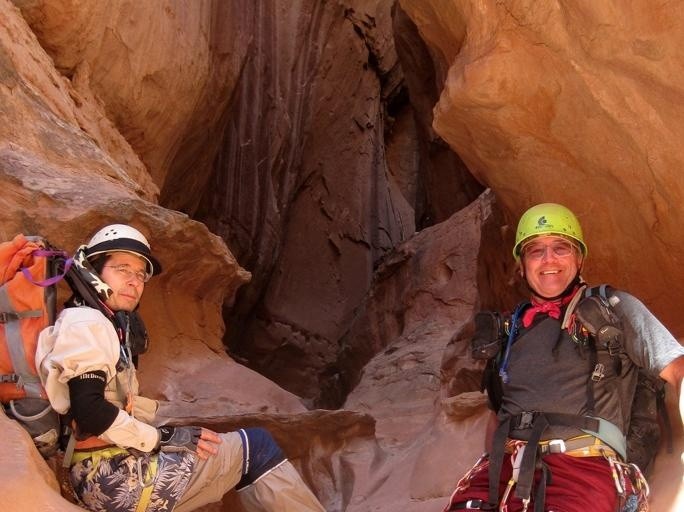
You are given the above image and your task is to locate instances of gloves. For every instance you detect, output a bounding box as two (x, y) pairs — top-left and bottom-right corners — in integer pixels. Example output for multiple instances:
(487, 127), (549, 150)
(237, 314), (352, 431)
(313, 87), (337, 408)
(153, 424), (202, 458)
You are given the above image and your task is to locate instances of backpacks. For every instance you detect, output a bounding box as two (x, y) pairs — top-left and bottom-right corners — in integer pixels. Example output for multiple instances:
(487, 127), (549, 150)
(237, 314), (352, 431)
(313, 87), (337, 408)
(469, 284), (674, 488)
(1, 232), (149, 461)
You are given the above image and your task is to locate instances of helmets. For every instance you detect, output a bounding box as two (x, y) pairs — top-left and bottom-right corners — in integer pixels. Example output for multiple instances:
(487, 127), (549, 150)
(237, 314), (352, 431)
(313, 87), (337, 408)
(511, 202), (588, 265)
(84, 223), (162, 283)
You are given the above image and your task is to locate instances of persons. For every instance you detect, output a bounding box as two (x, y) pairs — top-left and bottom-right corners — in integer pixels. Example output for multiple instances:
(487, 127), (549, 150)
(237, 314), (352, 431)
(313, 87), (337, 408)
(440, 202), (684, 512)
(33, 223), (327, 511)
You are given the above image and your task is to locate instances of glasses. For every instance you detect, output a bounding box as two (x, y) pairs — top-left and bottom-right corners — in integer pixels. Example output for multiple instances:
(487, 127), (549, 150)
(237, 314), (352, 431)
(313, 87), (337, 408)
(520, 241), (578, 259)
